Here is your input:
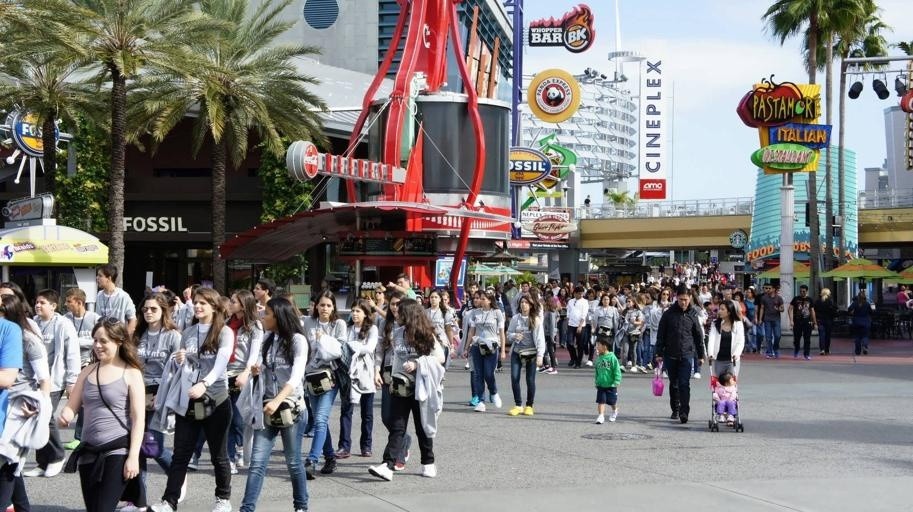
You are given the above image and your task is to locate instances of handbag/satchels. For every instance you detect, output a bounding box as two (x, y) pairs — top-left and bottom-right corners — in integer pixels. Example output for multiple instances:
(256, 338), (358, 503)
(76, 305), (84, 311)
(188, 392), (214, 420)
(379, 366), (392, 385)
(140, 431), (164, 459)
(389, 372), (416, 398)
(518, 347), (537, 365)
(262, 396), (303, 428)
(598, 325), (611, 336)
(478, 338), (498, 355)
(651, 377), (664, 396)
(304, 367), (337, 396)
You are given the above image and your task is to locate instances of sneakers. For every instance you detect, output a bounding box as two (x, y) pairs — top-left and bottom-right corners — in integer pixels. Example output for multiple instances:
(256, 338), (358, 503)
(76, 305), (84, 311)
(508, 406), (524, 416)
(404, 450), (409, 461)
(620, 365), (627, 373)
(421, 462), (437, 477)
(320, 458), (338, 474)
(670, 410), (679, 419)
(468, 396), (479, 406)
(524, 406), (534, 415)
(177, 472), (188, 504)
(22, 467), (45, 477)
(230, 461), (239, 475)
(393, 462), (404, 470)
(491, 392), (502, 409)
(150, 500), (174, 512)
(585, 360), (593, 367)
(120, 503), (147, 512)
(693, 372), (701, 379)
(718, 414), (726, 422)
(361, 448), (372, 457)
(595, 415), (604, 424)
(573, 362), (582, 369)
(568, 360), (575, 366)
(368, 463), (393, 481)
(335, 448), (351, 459)
(473, 402), (485, 412)
(680, 413), (688, 424)
(187, 453), (198, 471)
(629, 367), (638, 374)
(211, 496), (232, 512)
(727, 415), (734, 422)
(639, 366), (648, 374)
(608, 411), (618, 422)
(44, 456), (67, 478)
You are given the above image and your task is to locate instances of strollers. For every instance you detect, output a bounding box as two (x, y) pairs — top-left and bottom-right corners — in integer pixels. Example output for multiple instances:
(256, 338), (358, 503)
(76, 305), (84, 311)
(706, 356), (745, 433)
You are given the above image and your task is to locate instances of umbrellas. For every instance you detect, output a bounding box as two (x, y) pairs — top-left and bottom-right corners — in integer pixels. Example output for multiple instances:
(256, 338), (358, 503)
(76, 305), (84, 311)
(754, 260), (811, 279)
(885, 265), (913, 286)
(819, 256), (904, 294)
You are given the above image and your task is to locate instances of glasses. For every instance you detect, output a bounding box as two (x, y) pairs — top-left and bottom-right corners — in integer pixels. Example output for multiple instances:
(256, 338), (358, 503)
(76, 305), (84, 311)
(141, 307), (158, 313)
(98, 316), (119, 325)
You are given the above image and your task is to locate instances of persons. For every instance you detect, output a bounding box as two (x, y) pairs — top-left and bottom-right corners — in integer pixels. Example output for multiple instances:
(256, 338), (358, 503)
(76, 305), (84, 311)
(897, 285), (910, 311)
(787, 284), (818, 360)
(462, 288), (505, 411)
(656, 288), (703, 422)
(905, 285), (913, 299)
(460, 258), (786, 378)
(707, 301), (745, 426)
(848, 289), (876, 356)
(0, 264), (455, 511)
(594, 336), (621, 424)
(814, 288), (839, 356)
(506, 295), (546, 415)
